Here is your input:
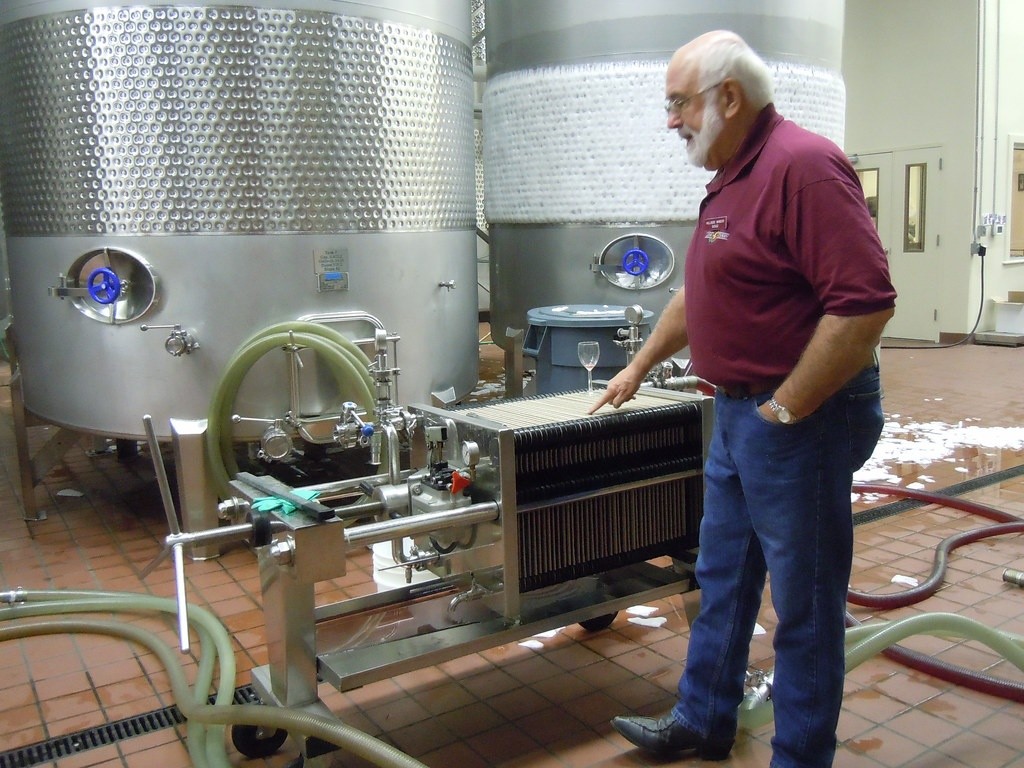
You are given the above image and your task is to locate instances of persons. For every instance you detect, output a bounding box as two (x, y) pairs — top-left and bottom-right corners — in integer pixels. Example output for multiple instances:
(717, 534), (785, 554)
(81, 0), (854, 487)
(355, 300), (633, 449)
(587, 31), (896, 768)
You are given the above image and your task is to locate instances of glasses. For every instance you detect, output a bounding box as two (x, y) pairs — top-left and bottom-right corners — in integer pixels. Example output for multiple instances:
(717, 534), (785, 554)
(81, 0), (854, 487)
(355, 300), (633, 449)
(663, 82), (721, 111)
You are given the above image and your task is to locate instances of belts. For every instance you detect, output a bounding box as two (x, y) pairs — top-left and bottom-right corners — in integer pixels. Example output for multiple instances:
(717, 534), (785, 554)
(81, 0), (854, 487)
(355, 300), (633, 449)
(714, 378), (790, 401)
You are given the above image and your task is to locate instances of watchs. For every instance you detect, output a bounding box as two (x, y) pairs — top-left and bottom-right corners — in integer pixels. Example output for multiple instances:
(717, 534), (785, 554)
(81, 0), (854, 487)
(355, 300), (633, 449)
(768, 396), (798, 425)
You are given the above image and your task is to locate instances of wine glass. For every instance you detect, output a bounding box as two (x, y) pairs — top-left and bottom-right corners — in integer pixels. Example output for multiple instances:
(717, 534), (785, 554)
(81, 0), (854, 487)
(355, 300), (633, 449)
(577, 340), (601, 396)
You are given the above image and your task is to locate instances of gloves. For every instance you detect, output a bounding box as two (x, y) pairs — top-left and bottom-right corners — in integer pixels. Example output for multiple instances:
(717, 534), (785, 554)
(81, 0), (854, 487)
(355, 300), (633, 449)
(252, 489), (321, 514)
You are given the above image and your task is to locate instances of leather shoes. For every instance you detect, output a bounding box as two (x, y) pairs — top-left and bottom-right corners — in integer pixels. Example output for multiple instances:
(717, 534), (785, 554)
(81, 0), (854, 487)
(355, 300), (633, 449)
(609, 712), (734, 763)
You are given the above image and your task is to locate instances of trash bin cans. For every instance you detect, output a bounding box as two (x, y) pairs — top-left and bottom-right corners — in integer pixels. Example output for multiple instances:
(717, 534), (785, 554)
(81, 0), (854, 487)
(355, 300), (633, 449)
(520, 301), (653, 396)
